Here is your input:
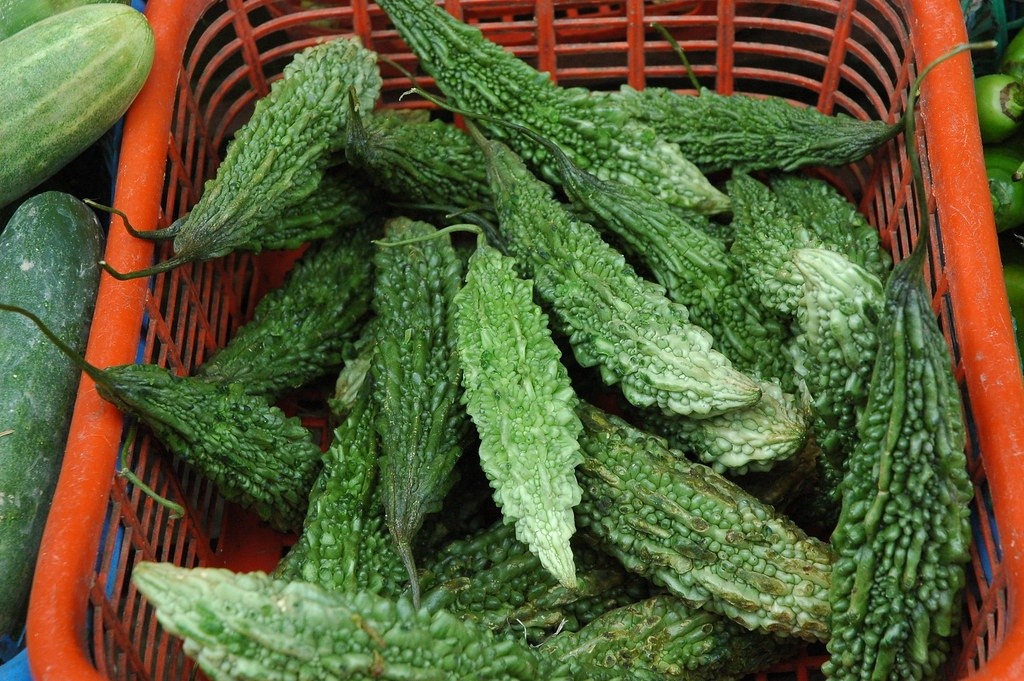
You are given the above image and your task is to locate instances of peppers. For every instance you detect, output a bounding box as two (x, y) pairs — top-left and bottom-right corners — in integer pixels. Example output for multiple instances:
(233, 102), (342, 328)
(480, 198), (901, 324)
(965, 23), (1024, 376)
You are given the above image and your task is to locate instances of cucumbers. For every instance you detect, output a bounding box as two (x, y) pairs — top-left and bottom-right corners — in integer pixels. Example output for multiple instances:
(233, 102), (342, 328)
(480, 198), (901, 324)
(0, 0), (168, 663)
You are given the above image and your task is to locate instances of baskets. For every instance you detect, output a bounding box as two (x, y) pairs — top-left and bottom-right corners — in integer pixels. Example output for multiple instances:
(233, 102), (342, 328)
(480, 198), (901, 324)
(25, 0), (1024, 681)
